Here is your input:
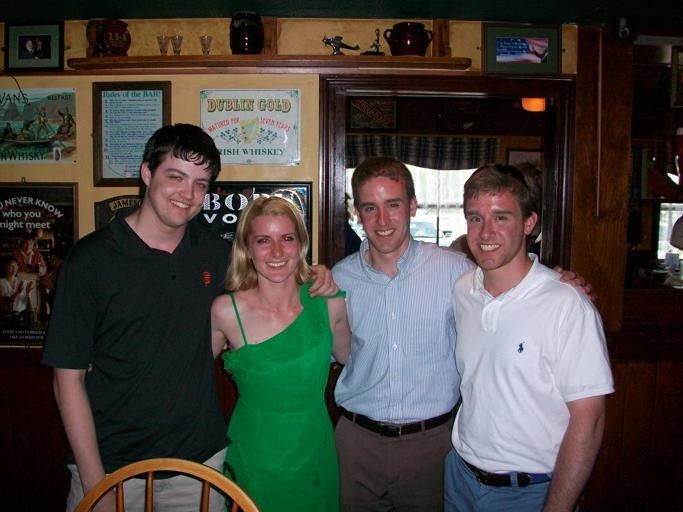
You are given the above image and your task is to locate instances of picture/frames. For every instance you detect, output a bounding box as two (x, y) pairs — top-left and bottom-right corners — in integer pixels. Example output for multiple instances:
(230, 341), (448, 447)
(481, 21), (561, 75)
(345, 95), (399, 131)
(504, 148), (541, 197)
(1, 182), (79, 349)
(3, 17), (63, 73)
(201, 180), (312, 272)
(91, 80), (172, 188)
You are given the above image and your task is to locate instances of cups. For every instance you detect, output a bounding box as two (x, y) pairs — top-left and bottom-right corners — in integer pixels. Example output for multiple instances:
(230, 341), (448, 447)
(156, 34), (170, 56)
(170, 35), (182, 55)
(200, 34), (212, 56)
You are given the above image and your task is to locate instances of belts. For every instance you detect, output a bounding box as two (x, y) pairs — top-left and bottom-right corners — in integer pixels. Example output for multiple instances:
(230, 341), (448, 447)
(340, 406), (455, 437)
(463, 460), (532, 487)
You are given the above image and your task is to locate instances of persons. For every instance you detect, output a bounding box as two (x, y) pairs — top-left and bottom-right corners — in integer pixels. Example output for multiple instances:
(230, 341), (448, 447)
(3, 106), (75, 147)
(40, 124), (340, 512)
(13, 235), (50, 330)
(33, 40), (45, 59)
(442, 162), (616, 511)
(20, 39), (39, 59)
(330, 155), (598, 511)
(0, 256), (36, 331)
(211, 196), (351, 512)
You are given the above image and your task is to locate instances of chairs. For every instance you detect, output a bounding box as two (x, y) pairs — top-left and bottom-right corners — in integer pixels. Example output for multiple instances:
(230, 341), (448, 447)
(69, 457), (263, 512)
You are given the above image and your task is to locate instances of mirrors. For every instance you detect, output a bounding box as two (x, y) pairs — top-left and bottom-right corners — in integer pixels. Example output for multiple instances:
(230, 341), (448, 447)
(317, 72), (571, 266)
